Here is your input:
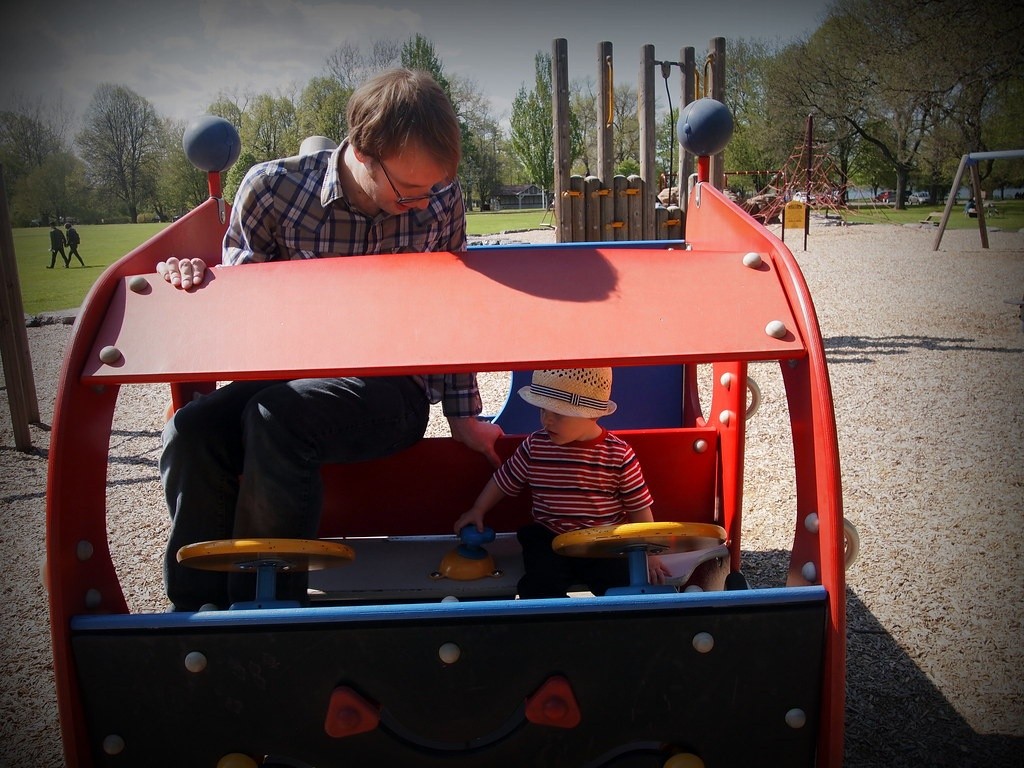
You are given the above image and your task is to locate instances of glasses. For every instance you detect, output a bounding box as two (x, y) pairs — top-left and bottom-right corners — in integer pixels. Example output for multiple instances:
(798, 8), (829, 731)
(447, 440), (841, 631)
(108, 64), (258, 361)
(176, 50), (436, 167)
(373, 152), (453, 203)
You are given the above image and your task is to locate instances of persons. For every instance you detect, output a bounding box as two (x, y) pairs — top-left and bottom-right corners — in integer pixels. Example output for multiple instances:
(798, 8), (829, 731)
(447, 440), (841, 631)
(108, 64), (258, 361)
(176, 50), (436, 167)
(882, 192), (889, 204)
(156, 69), (506, 616)
(38, 219), (41, 228)
(466, 195), (473, 210)
(453, 365), (672, 599)
(64, 222), (85, 266)
(47, 222), (69, 268)
(23, 220), (33, 228)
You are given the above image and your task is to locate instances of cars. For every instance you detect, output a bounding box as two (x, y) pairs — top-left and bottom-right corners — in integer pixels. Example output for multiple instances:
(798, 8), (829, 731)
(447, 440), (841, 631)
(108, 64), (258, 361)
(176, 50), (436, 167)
(793, 192), (816, 205)
(151, 215), (169, 223)
(171, 215), (180, 223)
(875, 191), (896, 203)
(904, 190), (912, 202)
(908, 192), (930, 206)
(44, 98), (862, 767)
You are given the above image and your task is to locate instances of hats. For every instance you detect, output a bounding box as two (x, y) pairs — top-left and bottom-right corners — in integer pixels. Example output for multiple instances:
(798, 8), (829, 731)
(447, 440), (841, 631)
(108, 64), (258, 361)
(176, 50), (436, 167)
(517, 366), (617, 419)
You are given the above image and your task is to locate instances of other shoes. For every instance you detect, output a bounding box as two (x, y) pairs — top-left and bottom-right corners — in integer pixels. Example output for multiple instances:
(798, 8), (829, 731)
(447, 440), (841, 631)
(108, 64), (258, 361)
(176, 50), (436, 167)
(62, 265), (69, 268)
(46, 265), (54, 269)
(81, 265), (85, 266)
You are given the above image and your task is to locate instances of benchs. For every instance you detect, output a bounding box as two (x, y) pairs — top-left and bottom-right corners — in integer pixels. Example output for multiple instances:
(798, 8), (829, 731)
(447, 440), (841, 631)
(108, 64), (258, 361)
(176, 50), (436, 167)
(233, 427), (723, 602)
